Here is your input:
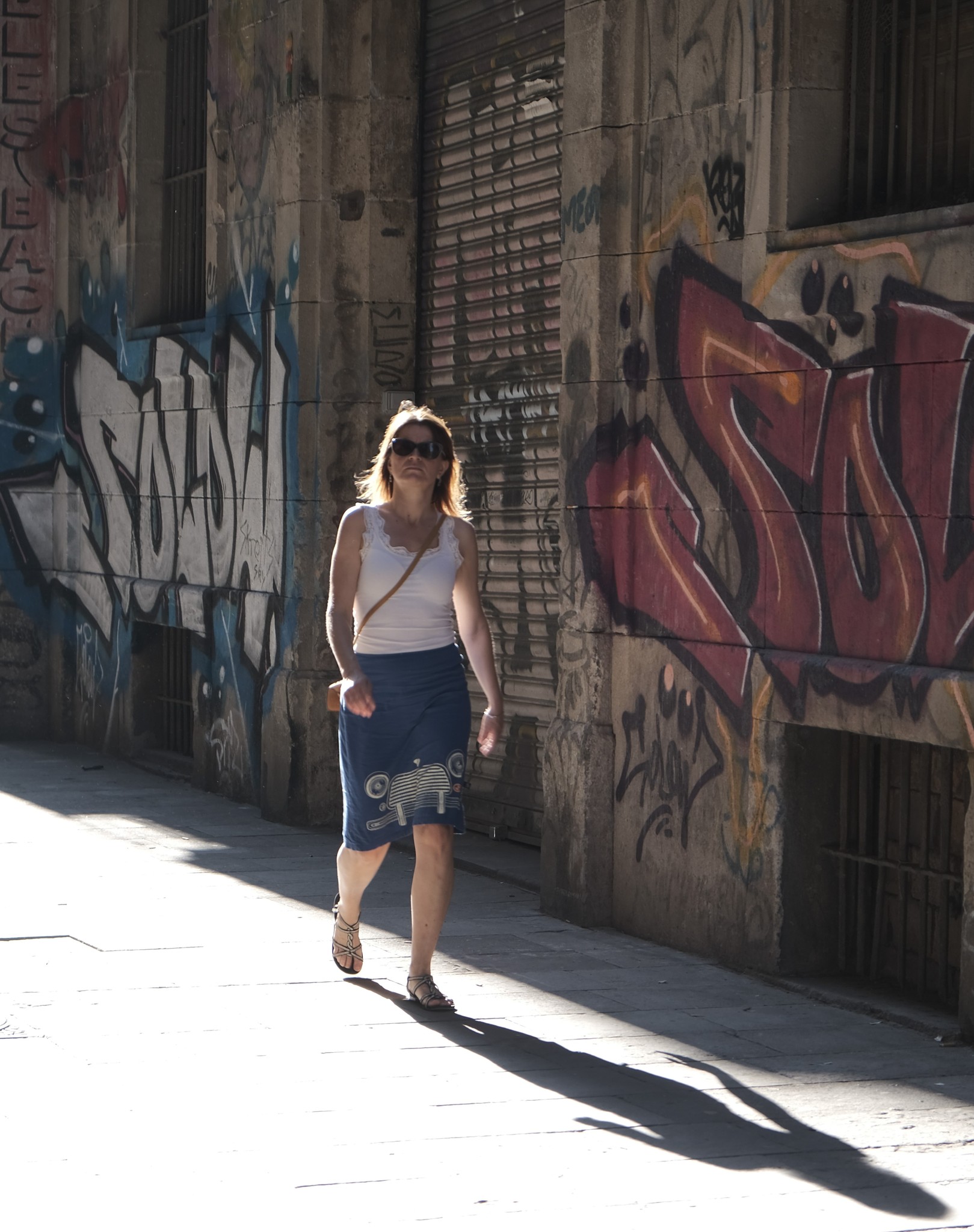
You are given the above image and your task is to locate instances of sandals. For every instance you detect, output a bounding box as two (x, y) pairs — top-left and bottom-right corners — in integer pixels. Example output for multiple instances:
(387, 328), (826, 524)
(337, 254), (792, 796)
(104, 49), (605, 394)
(332, 892), (363, 975)
(407, 974), (455, 1011)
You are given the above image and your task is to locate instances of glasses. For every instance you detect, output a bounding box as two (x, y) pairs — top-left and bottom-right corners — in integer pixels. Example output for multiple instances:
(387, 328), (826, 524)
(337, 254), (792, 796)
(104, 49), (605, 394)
(386, 437), (445, 460)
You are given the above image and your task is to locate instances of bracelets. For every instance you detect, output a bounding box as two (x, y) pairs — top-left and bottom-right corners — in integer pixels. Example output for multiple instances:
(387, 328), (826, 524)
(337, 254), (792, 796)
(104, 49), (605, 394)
(483, 708), (505, 718)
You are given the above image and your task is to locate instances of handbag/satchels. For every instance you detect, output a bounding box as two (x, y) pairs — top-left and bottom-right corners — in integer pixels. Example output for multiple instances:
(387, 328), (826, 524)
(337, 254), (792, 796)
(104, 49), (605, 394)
(325, 679), (343, 712)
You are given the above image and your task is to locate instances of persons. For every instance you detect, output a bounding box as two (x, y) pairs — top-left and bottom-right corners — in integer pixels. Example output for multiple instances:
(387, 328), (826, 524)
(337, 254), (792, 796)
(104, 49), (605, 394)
(326, 399), (503, 1012)
